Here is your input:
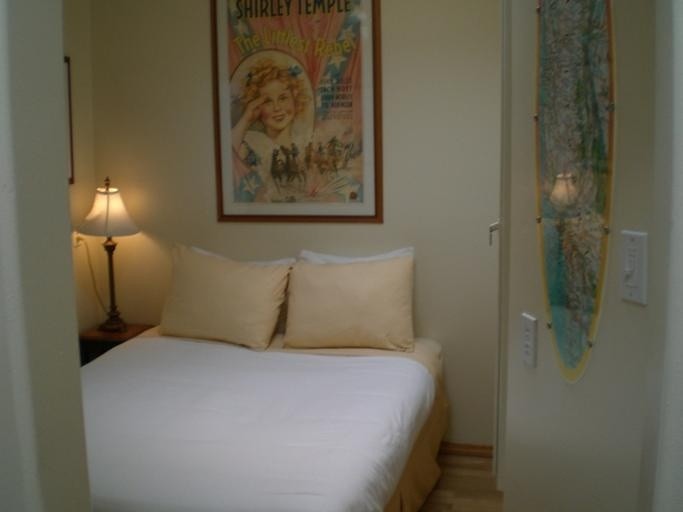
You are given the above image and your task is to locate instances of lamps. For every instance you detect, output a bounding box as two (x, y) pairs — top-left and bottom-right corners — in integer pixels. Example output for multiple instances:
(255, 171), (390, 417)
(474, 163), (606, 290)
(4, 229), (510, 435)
(76, 175), (140, 333)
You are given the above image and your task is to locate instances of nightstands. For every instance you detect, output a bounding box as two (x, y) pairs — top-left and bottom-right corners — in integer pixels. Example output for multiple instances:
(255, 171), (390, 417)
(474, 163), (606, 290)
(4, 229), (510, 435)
(79, 323), (156, 367)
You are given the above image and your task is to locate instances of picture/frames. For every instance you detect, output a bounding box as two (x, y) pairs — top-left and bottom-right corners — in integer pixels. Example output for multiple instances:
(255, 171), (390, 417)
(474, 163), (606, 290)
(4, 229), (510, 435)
(64, 54), (74, 184)
(533, 0), (615, 385)
(209, 0), (384, 224)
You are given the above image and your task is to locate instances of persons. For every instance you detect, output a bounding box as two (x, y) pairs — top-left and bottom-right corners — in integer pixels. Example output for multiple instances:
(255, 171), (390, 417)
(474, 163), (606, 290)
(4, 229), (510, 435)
(231, 64), (311, 201)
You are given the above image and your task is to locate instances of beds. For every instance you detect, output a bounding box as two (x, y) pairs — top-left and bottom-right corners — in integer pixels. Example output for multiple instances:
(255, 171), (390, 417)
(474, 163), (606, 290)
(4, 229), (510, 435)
(79, 314), (451, 512)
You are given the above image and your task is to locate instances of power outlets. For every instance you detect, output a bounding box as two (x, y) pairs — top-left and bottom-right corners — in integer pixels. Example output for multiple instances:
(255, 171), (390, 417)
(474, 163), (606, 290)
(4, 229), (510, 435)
(70, 224), (82, 247)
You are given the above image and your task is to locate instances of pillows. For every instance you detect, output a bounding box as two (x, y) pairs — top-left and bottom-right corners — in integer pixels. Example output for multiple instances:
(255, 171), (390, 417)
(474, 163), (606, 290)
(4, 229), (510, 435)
(155, 240), (416, 351)
